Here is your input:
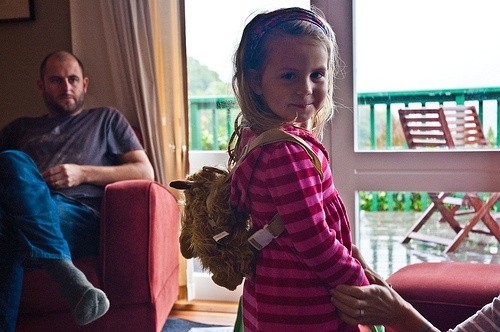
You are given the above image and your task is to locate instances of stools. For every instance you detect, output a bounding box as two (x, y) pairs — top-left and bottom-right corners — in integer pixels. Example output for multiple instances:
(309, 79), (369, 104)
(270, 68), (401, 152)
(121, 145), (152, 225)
(383, 262), (500, 332)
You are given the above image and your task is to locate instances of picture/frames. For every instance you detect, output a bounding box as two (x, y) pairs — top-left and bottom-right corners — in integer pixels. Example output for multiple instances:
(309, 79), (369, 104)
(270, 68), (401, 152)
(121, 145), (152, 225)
(0, 0), (36, 24)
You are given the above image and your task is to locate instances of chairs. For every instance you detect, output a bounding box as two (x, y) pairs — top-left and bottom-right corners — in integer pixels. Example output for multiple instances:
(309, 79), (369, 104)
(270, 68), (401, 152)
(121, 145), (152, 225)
(398, 104), (500, 255)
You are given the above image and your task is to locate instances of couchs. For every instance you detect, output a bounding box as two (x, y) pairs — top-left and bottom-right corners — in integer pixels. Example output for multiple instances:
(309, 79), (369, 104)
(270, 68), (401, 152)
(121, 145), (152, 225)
(15, 125), (179, 332)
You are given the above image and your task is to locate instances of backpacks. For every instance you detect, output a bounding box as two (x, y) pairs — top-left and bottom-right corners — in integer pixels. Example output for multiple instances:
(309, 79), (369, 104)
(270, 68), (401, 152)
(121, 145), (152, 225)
(168, 128), (324, 291)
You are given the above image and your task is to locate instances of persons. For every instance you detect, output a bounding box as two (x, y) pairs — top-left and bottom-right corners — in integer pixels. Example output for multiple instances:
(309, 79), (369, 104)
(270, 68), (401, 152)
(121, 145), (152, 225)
(234, 7), (375, 331)
(332, 242), (500, 331)
(1, 49), (160, 330)
